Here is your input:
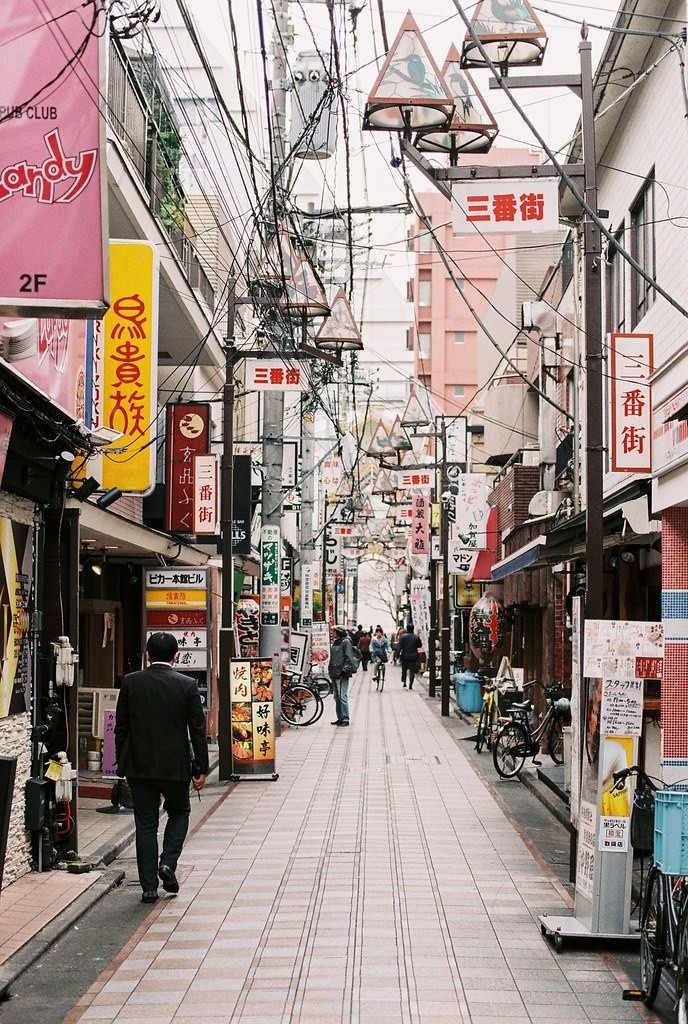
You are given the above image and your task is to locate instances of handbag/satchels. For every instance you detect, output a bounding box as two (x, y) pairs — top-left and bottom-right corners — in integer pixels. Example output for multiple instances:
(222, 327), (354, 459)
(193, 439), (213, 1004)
(399, 650), (406, 661)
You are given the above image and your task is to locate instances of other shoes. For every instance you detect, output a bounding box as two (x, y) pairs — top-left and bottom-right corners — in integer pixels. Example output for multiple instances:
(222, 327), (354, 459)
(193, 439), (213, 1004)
(142, 890), (159, 904)
(371, 674), (377, 680)
(409, 685), (412, 689)
(159, 864), (179, 893)
(330, 720), (342, 725)
(340, 721), (349, 726)
(403, 684), (406, 687)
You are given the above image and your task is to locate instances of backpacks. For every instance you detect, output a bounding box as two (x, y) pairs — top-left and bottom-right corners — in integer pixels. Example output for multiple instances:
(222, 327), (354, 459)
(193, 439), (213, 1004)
(341, 638), (362, 672)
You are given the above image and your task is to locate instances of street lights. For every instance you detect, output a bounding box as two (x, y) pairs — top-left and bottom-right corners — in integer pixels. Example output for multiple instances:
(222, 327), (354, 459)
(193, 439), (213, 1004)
(361, 0), (607, 885)
(368, 387), (449, 717)
(215, 248), (366, 783)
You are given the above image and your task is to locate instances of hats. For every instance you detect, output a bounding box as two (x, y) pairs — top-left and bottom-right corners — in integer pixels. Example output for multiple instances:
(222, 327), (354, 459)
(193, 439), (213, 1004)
(330, 624), (347, 634)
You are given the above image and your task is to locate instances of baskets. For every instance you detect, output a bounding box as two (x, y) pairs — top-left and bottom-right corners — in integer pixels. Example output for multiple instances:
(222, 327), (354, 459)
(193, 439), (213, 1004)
(653, 790), (688, 876)
(374, 651), (388, 663)
(544, 685), (573, 713)
(631, 800), (654, 850)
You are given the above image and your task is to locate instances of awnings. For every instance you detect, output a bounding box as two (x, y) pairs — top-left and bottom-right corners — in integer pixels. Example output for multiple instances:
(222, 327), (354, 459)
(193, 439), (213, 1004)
(490, 495), (656, 583)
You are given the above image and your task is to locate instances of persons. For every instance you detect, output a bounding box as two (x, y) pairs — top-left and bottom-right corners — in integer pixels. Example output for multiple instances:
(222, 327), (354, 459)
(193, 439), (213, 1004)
(328, 625), (356, 727)
(395, 624), (422, 690)
(369, 628), (390, 681)
(358, 631), (371, 671)
(355, 624), (383, 664)
(114, 632), (209, 904)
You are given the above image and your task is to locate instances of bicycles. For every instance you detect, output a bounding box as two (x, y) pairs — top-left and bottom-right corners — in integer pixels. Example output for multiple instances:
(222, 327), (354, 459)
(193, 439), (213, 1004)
(491, 678), (572, 783)
(371, 650), (392, 693)
(609, 765), (688, 1023)
(278, 661), (331, 729)
(453, 652), (469, 695)
(473, 674), (515, 753)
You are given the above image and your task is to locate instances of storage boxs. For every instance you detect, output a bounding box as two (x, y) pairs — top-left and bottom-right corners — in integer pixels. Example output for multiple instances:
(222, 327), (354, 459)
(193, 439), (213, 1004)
(452, 672), (482, 712)
(654, 791), (688, 876)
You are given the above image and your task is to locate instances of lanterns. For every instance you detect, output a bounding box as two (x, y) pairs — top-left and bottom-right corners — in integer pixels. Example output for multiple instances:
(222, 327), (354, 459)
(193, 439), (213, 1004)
(469, 591), (507, 664)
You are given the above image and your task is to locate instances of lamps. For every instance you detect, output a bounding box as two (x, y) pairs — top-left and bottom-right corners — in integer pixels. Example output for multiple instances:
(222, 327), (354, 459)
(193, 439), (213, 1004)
(574, 558), (586, 579)
(609, 545), (651, 567)
(28, 451), (76, 475)
(79, 542), (108, 576)
(56, 486), (122, 510)
(24, 466), (99, 504)
(127, 562), (138, 584)
(458, 530), (502, 544)
